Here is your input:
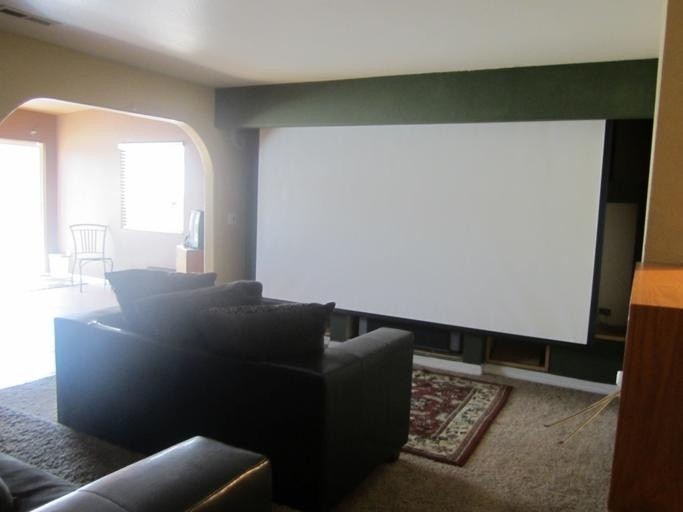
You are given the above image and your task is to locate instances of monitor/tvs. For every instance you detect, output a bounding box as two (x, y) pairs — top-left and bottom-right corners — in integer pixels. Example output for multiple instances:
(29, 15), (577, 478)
(186, 209), (204, 250)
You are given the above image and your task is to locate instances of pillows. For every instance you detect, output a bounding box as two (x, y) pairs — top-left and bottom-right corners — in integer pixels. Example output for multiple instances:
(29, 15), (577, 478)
(105, 270), (336, 363)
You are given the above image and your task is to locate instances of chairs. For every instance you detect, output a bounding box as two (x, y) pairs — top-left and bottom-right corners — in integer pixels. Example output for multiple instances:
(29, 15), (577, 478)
(69, 224), (113, 296)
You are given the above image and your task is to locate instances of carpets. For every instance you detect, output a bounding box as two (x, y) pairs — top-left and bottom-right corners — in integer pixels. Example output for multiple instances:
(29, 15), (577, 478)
(400, 363), (514, 468)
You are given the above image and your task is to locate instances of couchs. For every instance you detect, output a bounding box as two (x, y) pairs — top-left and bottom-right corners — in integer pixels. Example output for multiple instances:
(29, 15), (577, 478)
(54, 306), (414, 512)
(0, 436), (271, 512)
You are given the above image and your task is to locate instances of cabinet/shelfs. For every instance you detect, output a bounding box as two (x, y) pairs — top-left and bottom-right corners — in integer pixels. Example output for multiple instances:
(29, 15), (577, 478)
(176, 245), (204, 272)
